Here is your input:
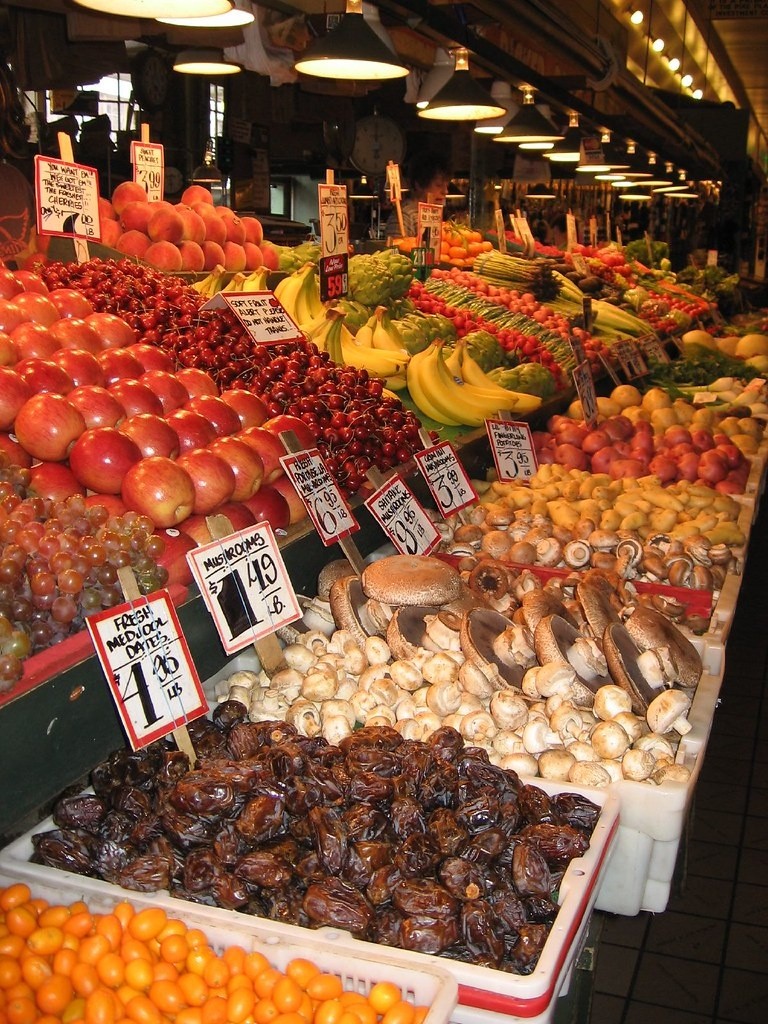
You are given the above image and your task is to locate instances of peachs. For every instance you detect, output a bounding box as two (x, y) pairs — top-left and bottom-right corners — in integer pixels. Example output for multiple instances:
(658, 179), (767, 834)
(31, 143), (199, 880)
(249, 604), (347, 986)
(93, 181), (279, 273)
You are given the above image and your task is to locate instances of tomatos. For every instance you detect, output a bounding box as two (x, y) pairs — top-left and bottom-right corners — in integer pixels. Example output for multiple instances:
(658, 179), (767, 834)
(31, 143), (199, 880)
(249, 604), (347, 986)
(0, 883), (432, 1024)
(571, 243), (703, 318)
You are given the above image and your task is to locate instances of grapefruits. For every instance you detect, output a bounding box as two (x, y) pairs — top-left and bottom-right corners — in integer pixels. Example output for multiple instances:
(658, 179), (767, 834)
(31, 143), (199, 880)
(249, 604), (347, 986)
(682, 330), (768, 372)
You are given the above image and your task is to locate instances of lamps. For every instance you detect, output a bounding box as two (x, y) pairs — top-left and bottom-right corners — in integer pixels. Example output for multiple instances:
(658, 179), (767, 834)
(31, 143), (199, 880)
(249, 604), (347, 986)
(82, 0), (701, 201)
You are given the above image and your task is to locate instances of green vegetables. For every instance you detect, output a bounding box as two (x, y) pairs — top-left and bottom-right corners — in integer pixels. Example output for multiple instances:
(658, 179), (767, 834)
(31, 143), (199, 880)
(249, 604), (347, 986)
(648, 342), (767, 383)
(623, 240), (744, 303)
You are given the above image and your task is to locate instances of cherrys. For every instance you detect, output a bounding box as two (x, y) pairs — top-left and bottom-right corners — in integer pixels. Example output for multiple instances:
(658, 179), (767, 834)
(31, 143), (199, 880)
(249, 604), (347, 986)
(30, 257), (446, 499)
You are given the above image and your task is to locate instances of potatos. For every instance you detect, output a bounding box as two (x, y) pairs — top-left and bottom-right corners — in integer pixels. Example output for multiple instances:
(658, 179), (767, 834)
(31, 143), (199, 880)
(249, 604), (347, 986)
(466, 384), (763, 548)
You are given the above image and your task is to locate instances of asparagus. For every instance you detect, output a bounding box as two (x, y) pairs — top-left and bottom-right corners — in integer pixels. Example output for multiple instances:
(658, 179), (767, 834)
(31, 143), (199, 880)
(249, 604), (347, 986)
(423, 248), (587, 377)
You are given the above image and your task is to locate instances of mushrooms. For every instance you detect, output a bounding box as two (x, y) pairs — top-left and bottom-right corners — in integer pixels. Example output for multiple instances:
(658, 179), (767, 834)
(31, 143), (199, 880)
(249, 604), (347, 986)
(215, 503), (742, 790)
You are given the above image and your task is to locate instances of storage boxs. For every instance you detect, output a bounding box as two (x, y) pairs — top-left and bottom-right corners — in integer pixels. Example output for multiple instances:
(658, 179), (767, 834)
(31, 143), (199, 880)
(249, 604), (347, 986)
(2, 228), (768, 1024)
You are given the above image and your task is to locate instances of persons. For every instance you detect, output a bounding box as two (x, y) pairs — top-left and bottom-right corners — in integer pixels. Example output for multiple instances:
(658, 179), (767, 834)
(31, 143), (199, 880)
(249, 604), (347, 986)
(386, 154), (450, 241)
(492, 198), (639, 247)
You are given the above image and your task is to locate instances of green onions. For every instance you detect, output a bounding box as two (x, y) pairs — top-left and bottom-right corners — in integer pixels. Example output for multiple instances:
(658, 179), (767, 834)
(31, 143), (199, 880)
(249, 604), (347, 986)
(643, 377), (768, 416)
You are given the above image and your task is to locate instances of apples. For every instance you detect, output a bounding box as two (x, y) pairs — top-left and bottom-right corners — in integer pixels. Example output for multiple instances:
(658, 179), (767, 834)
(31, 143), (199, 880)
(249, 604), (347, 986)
(0, 263), (317, 585)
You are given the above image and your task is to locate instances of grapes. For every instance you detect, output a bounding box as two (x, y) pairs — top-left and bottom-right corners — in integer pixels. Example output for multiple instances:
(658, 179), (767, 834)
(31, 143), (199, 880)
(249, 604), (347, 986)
(590, 264), (678, 335)
(0, 450), (167, 698)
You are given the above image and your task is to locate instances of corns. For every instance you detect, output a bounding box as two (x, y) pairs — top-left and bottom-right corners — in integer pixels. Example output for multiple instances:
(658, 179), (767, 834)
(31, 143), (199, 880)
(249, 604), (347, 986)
(551, 271), (655, 344)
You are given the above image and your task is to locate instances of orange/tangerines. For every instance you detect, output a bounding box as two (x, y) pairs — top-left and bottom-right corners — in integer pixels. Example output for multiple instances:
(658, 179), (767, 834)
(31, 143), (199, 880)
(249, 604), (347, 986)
(392, 222), (493, 268)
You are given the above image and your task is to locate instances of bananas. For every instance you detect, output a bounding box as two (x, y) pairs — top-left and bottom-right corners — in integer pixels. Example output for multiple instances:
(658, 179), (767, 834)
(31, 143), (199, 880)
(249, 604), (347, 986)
(189, 263), (543, 427)
